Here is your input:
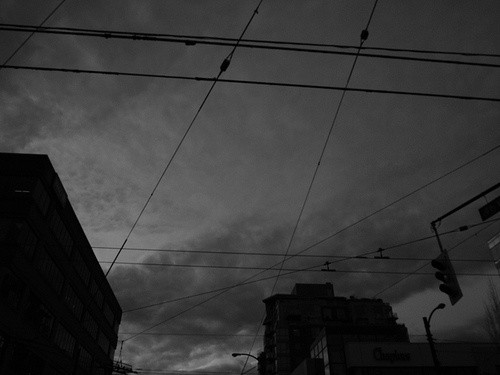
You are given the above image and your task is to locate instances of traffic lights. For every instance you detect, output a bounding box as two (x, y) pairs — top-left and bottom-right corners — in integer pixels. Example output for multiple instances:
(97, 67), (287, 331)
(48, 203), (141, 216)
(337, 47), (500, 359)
(432, 248), (462, 306)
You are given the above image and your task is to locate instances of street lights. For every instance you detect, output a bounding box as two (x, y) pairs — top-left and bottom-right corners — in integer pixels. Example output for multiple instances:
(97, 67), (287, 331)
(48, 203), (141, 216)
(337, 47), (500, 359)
(422, 303), (447, 375)
(232, 353), (263, 375)
